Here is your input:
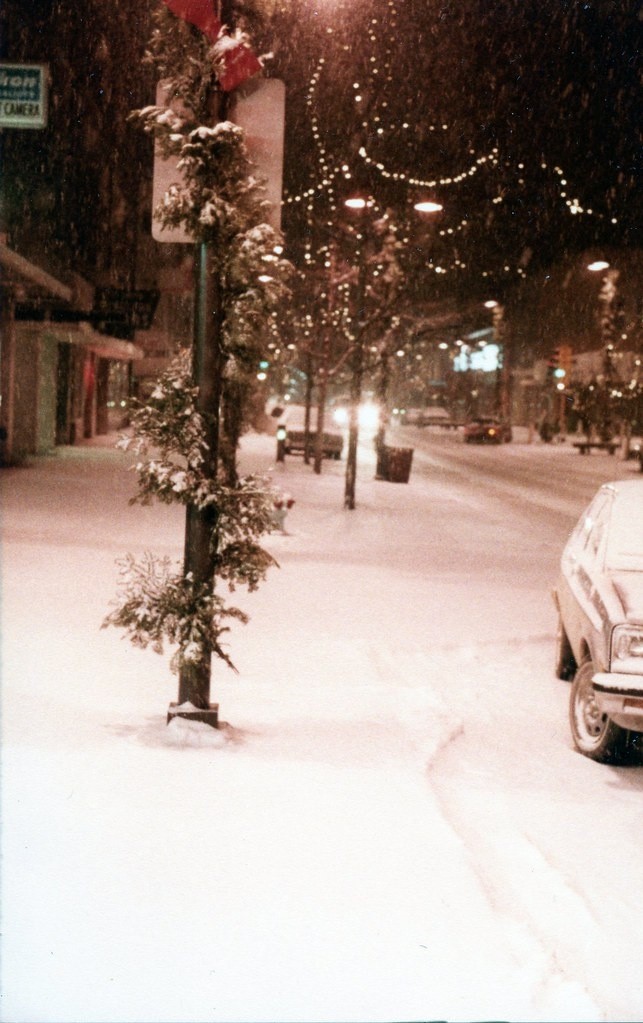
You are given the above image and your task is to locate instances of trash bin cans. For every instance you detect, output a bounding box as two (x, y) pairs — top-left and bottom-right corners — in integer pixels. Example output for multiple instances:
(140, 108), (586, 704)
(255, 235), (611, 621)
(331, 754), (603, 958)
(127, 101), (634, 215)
(375, 446), (414, 484)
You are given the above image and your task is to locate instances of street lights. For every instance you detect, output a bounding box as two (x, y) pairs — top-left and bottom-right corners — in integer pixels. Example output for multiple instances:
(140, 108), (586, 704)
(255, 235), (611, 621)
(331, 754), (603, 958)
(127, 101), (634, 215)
(298, 156), (371, 463)
(344, 167), (445, 511)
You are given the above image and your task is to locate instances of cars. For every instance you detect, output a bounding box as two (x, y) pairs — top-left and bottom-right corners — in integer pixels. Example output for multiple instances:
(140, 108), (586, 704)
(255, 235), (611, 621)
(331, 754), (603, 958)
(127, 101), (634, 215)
(400, 408), (422, 426)
(275, 405), (345, 462)
(417, 406), (452, 431)
(549, 480), (643, 764)
(462, 414), (512, 445)
